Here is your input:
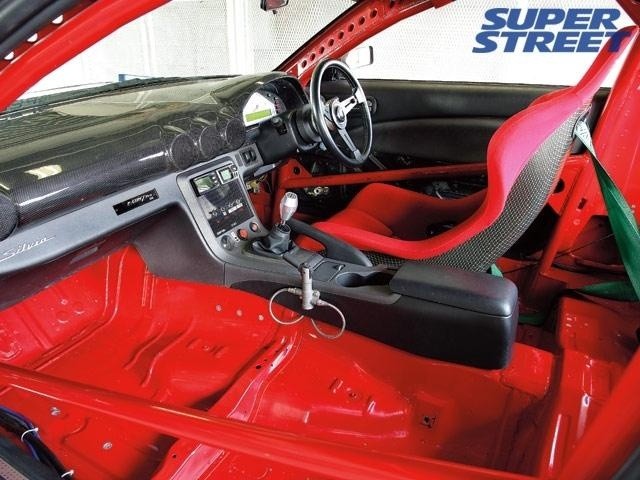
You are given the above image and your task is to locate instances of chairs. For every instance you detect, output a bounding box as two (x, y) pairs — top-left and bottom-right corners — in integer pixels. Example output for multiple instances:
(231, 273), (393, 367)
(298, 24), (639, 277)
